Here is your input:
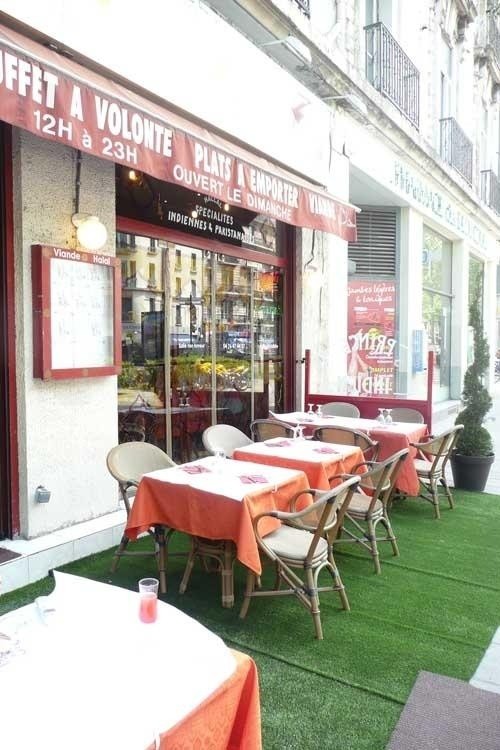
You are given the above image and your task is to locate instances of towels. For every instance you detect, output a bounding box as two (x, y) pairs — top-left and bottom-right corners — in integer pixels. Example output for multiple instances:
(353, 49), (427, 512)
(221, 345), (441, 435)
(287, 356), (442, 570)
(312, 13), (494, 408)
(264, 440), (290, 448)
(239, 475), (268, 485)
(178, 465), (211, 475)
(311, 447), (338, 455)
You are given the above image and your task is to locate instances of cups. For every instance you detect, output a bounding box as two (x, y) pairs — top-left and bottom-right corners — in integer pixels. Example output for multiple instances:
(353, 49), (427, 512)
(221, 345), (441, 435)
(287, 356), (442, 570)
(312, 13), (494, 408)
(136, 574), (161, 628)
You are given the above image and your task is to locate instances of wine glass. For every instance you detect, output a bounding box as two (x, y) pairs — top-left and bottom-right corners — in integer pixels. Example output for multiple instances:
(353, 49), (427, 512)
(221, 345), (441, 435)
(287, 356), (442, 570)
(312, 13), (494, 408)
(384, 407), (394, 426)
(288, 425), (308, 448)
(377, 406), (387, 425)
(315, 404), (326, 417)
(306, 400), (316, 417)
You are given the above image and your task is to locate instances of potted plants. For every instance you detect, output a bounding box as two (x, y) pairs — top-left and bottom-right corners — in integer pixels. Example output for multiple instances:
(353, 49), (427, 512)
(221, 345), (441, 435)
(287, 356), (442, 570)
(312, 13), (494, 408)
(449, 277), (496, 492)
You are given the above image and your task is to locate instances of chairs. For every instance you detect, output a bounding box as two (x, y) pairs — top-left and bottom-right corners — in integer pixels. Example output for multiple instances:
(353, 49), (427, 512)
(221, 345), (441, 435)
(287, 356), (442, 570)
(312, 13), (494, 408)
(378, 407), (425, 426)
(326, 447), (411, 570)
(311, 424), (381, 472)
(315, 399), (361, 420)
(249, 417), (299, 444)
(237, 474), (359, 639)
(107, 439), (180, 592)
(408, 423), (465, 518)
(203, 425), (256, 459)
